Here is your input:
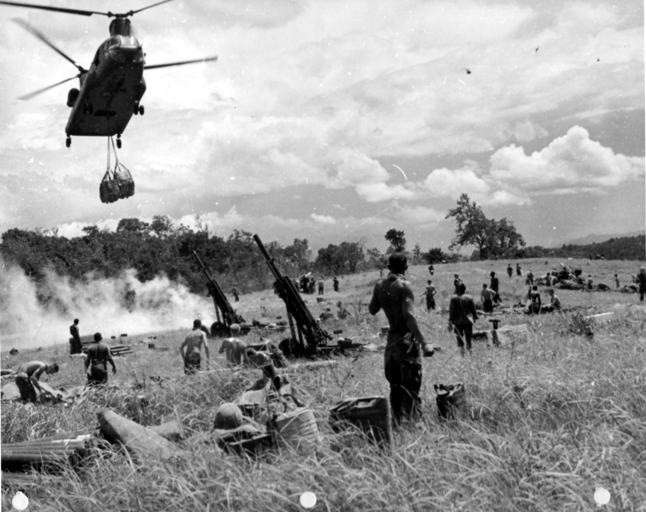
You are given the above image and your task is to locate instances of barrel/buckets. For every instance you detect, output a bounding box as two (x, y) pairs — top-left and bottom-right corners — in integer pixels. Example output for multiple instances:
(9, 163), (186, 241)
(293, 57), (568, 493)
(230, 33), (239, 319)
(437, 384), (468, 420)
(276, 410), (322, 455)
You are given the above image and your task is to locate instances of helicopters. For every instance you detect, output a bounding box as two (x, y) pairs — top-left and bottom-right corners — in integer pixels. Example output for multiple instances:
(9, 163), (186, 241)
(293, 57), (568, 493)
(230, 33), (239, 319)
(0, 0), (218, 149)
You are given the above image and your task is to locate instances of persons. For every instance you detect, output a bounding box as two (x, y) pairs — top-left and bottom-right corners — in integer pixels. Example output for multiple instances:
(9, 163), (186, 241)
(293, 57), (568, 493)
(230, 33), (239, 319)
(598, 265), (645, 302)
(366, 254), (435, 429)
(14, 318), (118, 404)
(313, 272), (352, 320)
(423, 264), (503, 353)
(180, 317), (285, 429)
(506, 262), (593, 314)
